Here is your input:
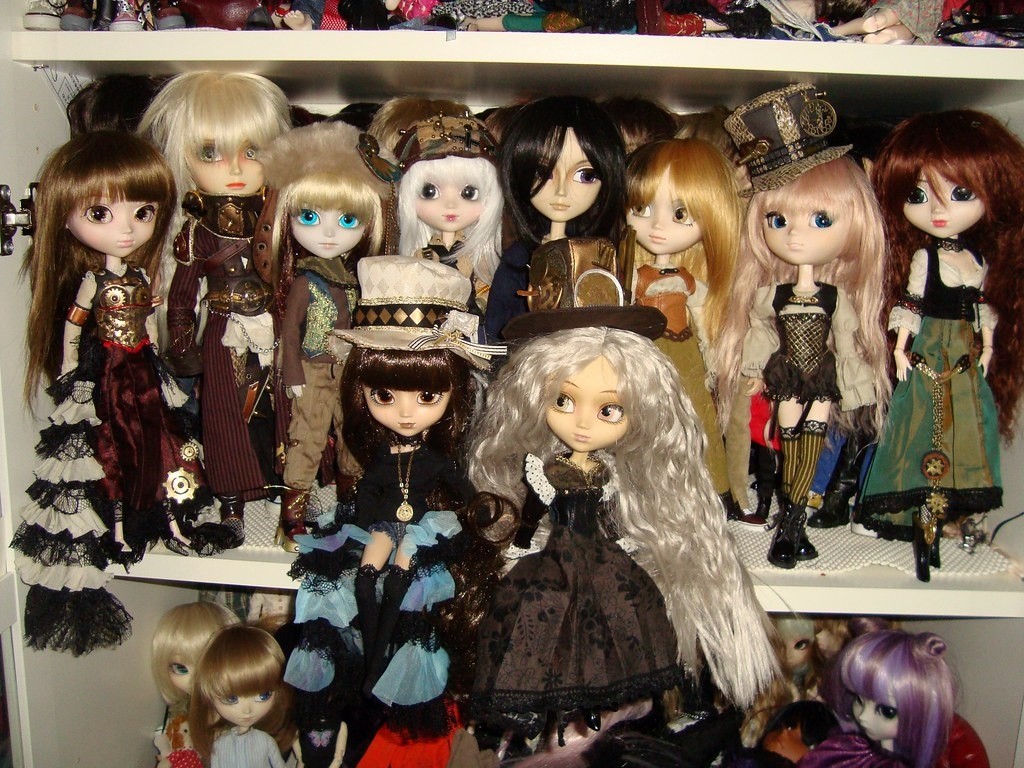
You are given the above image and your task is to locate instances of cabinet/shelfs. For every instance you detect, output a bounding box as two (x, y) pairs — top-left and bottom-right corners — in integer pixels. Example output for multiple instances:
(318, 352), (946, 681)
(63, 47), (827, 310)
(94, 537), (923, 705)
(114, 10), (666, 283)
(0, 0), (1024, 768)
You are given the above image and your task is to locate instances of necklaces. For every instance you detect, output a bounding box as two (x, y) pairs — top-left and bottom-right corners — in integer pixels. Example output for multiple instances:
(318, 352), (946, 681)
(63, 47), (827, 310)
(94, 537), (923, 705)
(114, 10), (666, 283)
(397, 442), (414, 523)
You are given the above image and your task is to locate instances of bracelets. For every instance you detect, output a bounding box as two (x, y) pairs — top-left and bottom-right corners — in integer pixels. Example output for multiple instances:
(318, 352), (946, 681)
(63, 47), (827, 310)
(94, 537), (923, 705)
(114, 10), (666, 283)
(894, 348), (904, 352)
(982, 345), (995, 351)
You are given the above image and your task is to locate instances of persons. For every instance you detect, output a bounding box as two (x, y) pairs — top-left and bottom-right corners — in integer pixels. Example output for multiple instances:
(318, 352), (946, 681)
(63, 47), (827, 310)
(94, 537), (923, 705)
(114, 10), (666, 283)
(17, 69), (292, 555)
(503, 100), (749, 530)
(852, 107), (1024, 582)
(258, 122), (388, 554)
(57, 0), (946, 48)
(462, 325), (782, 735)
(395, 108), (501, 317)
(714, 614), (992, 768)
(715, 157), (893, 571)
(143, 598), (305, 768)
(330, 253), (519, 706)
